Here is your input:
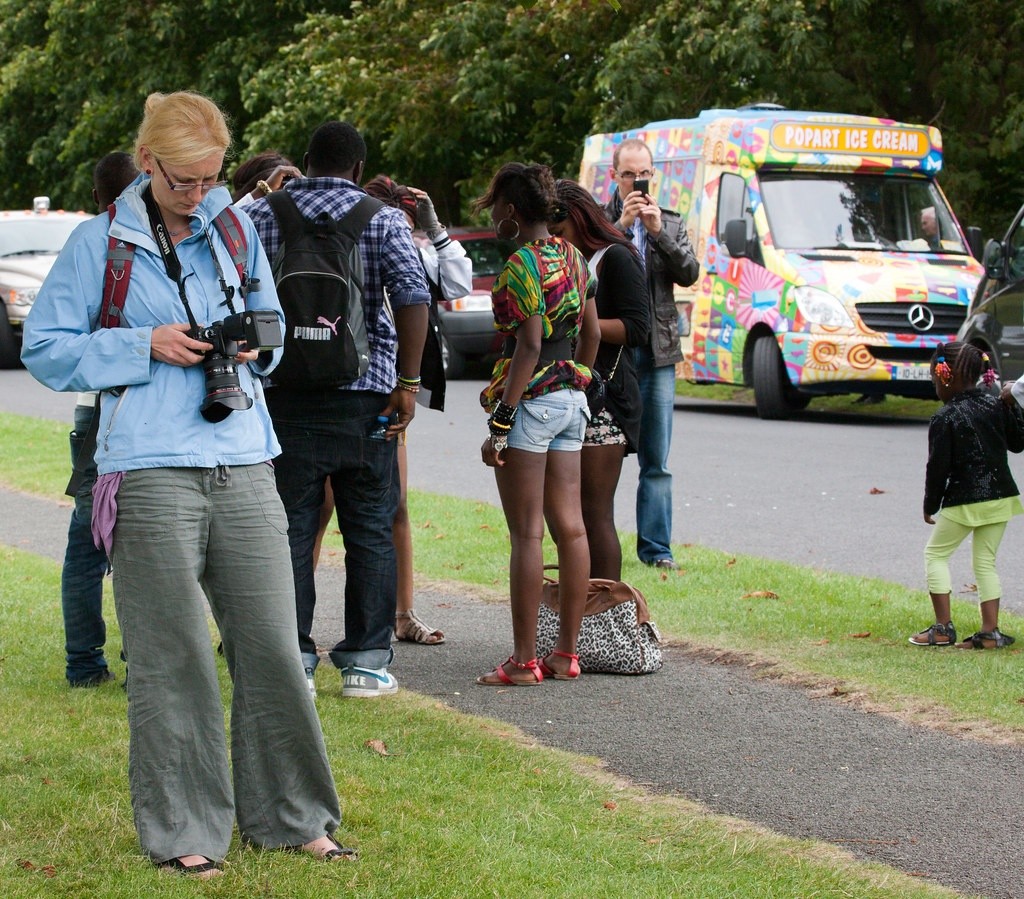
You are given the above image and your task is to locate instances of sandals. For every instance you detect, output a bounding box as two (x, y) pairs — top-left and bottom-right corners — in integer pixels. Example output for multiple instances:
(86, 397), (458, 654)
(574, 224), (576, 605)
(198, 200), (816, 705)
(294, 834), (361, 864)
(537, 648), (580, 681)
(954, 627), (1003, 649)
(475, 657), (543, 687)
(156, 853), (224, 877)
(907, 620), (957, 646)
(394, 610), (444, 643)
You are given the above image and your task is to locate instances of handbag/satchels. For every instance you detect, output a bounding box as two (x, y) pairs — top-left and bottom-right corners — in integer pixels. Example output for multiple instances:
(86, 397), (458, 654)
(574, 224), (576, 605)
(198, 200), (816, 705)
(534, 564), (662, 674)
(585, 368), (606, 421)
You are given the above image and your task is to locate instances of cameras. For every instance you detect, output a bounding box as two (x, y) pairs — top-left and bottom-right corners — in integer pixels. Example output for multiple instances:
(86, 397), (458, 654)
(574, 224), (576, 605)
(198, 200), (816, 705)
(182, 310), (283, 423)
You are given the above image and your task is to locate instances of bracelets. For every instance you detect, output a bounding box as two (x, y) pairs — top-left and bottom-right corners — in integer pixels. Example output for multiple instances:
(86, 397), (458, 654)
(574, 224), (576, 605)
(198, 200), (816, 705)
(487, 399), (519, 451)
(396, 372), (421, 392)
(255, 180), (272, 195)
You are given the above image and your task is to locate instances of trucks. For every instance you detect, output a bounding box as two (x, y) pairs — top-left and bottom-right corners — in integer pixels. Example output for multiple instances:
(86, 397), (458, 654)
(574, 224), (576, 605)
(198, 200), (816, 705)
(577, 102), (983, 418)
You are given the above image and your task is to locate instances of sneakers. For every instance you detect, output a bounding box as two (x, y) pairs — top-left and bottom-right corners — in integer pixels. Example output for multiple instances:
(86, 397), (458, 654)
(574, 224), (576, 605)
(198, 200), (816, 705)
(340, 662), (398, 697)
(306, 677), (316, 698)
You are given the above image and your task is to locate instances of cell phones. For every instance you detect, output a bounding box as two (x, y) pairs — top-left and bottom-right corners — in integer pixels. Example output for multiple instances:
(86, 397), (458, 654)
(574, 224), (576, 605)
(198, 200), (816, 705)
(633, 179), (649, 206)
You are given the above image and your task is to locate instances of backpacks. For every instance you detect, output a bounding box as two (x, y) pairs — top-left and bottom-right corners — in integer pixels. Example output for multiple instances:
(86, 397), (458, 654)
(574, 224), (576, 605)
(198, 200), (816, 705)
(264, 189), (387, 392)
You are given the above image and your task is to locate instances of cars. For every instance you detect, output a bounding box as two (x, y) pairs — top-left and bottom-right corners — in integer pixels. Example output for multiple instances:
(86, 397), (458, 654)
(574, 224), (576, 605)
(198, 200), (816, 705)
(952, 202), (1024, 382)
(438, 226), (502, 381)
(0, 196), (97, 370)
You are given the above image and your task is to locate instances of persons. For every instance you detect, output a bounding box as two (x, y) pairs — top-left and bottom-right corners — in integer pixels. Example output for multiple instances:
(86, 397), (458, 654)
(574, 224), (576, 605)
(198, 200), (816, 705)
(243, 120), (432, 698)
(600, 139), (700, 570)
(467, 161), (602, 687)
(850, 391), (886, 406)
(235, 165), (474, 646)
(61, 150), (298, 690)
(545, 181), (652, 583)
(920, 206), (941, 252)
(19, 91), (359, 879)
(909, 341), (1024, 652)
(1000, 373), (1024, 410)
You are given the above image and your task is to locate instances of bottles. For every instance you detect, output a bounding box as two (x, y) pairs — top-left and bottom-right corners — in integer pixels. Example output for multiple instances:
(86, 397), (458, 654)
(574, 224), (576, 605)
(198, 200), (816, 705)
(369, 415), (390, 440)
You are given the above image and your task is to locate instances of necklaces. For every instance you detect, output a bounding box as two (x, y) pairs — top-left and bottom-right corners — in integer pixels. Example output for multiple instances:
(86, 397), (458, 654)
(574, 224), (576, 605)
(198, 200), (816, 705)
(168, 224), (188, 236)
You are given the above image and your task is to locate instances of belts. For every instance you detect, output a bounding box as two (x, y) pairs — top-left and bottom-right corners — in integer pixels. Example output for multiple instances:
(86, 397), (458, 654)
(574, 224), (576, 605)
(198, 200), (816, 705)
(78, 393), (97, 407)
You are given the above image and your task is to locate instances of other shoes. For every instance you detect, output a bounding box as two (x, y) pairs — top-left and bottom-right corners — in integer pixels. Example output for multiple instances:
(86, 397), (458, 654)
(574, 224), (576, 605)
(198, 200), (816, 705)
(656, 559), (679, 569)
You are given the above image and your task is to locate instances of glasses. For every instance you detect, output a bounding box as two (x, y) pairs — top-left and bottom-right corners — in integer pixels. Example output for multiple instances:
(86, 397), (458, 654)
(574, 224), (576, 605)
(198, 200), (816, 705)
(614, 168), (655, 183)
(154, 156), (228, 191)
